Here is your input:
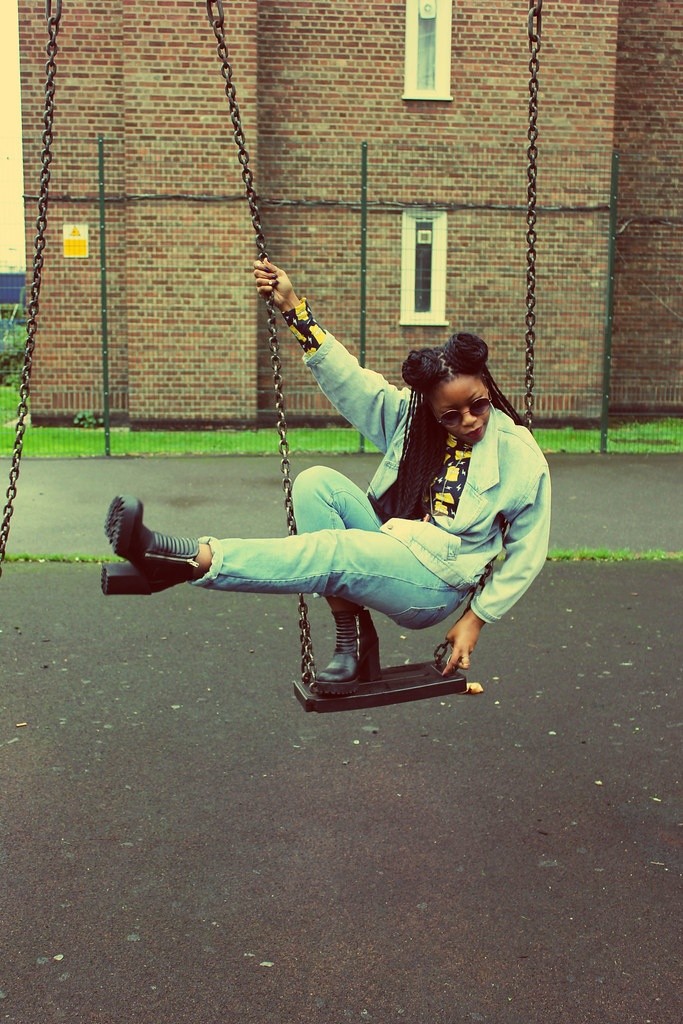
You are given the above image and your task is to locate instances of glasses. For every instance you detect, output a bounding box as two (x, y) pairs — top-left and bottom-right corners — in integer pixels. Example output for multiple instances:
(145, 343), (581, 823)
(427, 373), (492, 427)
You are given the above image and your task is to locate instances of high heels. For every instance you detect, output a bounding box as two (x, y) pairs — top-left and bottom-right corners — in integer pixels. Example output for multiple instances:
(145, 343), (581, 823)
(312, 609), (382, 695)
(101, 495), (200, 595)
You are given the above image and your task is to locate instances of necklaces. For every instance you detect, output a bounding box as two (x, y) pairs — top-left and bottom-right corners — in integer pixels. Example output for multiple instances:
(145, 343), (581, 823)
(424, 445), (468, 522)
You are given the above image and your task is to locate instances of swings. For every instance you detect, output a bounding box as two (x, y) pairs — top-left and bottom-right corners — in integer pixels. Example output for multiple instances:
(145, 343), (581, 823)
(0, 0), (65, 574)
(204, 0), (547, 717)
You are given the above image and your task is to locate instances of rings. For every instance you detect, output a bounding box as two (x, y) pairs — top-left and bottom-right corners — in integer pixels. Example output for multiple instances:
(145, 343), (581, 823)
(462, 656), (470, 659)
(462, 663), (471, 666)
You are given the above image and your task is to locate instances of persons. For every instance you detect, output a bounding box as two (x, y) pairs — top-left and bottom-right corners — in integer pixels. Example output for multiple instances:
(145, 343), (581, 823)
(99, 258), (551, 696)
